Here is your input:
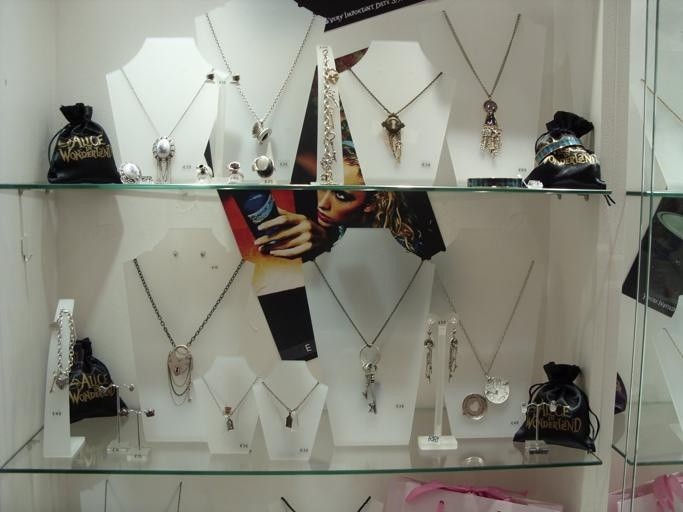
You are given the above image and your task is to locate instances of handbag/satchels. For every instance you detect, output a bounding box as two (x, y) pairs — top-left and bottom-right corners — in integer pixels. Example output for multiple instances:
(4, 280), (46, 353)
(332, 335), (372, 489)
(385, 474), (566, 512)
(607, 472), (683, 512)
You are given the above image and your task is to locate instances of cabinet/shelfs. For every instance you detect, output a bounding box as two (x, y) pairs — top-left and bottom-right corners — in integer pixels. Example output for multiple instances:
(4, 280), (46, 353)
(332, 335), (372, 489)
(0, 182), (612, 477)
(609, 190), (683, 468)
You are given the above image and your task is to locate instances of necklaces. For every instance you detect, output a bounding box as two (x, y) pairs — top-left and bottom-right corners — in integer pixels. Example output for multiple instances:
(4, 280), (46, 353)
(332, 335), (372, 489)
(196, 373), (257, 431)
(310, 255), (427, 415)
(132, 256), (247, 407)
(441, 8), (521, 157)
(260, 379), (320, 428)
(342, 61), (444, 162)
(203, 9), (317, 132)
(50, 307), (76, 393)
(434, 261), (536, 407)
(118, 59), (215, 164)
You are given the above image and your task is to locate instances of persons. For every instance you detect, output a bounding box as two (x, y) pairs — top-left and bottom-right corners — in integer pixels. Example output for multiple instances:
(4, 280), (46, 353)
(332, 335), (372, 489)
(254, 117), (430, 260)
